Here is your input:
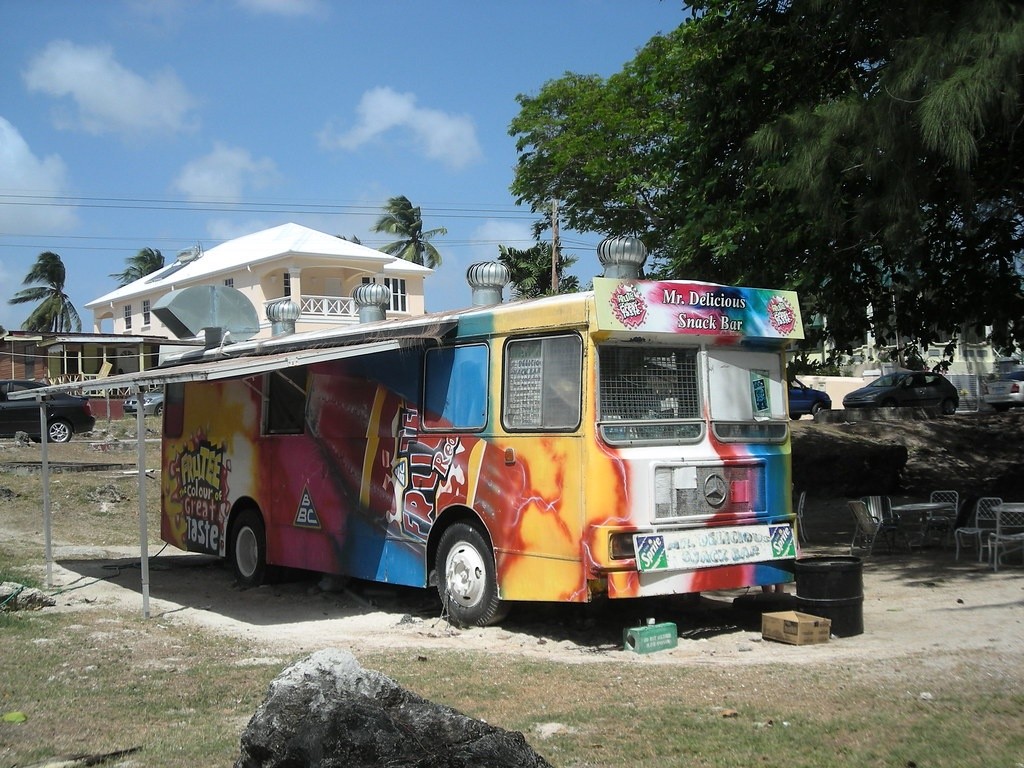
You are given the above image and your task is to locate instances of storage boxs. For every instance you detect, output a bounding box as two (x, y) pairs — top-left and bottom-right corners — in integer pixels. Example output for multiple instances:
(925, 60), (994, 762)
(762, 610), (832, 646)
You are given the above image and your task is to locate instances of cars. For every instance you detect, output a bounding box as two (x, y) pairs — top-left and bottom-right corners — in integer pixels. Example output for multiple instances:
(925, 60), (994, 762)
(0, 379), (97, 444)
(983, 355), (1024, 412)
(788, 376), (832, 421)
(121, 383), (165, 417)
(841, 369), (960, 415)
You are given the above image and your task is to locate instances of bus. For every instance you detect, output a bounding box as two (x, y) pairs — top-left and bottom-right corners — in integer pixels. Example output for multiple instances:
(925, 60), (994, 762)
(152, 239), (808, 629)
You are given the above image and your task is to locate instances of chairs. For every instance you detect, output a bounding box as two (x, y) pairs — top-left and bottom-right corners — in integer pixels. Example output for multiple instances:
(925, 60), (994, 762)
(857, 496), (901, 550)
(921, 489), (959, 552)
(797, 491), (809, 543)
(954, 497), (1008, 564)
(920, 497), (976, 554)
(987, 502), (1024, 570)
(847, 500), (911, 561)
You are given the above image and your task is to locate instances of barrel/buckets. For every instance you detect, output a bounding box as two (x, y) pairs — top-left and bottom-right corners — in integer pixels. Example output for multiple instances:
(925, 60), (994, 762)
(791, 554), (864, 638)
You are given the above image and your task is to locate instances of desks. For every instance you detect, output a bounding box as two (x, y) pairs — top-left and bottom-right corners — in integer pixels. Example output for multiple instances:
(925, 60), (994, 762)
(991, 505), (1024, 573)
(891, 502), (955, 554)
